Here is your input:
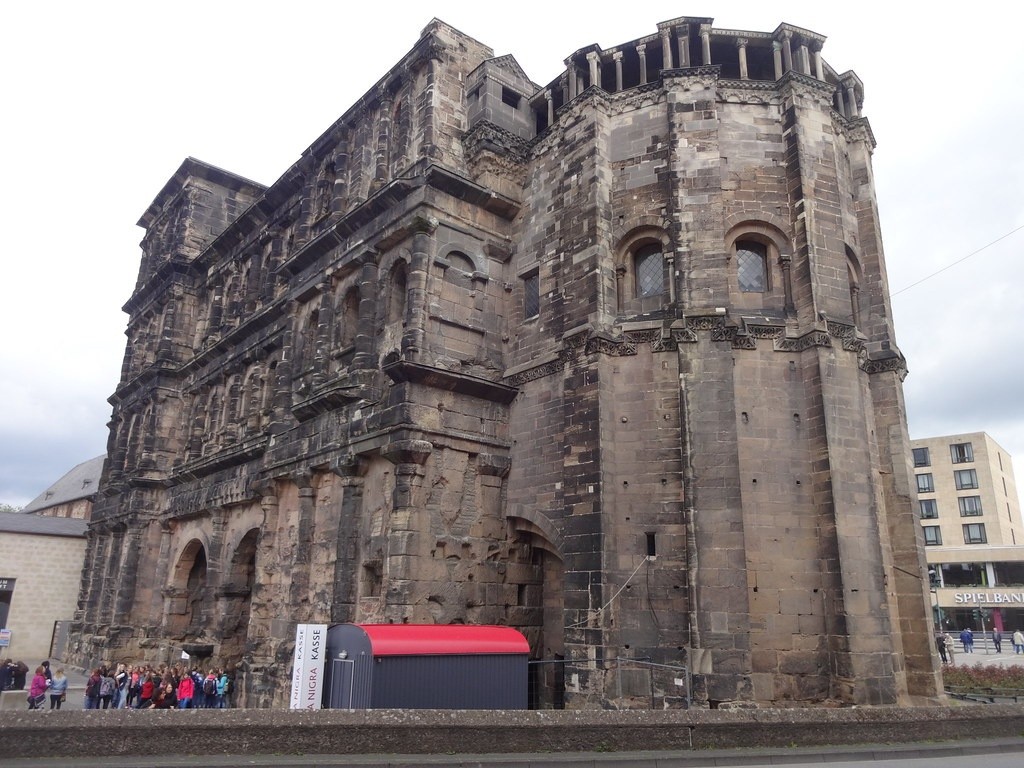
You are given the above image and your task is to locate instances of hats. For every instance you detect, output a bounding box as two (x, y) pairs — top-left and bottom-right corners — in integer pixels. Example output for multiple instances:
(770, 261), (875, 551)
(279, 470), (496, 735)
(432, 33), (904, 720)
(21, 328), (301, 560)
(56, 667), (65, 673)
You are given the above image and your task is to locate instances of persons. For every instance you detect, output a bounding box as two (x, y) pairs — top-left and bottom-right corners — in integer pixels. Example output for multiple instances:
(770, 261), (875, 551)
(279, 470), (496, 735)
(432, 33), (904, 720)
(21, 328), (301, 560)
(85, 662), (235, 709)
(936, 632), (955, 667)
(959, 628), (974, 653)
(0, 657), (27, 691)
(27, 661), (69, 709)
(992, 627), (1001, 653)
(1009, 629), (1024, 654)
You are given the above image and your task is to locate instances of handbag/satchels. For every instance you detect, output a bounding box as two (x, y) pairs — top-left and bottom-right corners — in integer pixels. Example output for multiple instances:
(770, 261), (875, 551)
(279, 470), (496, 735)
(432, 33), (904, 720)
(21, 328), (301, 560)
(59, 689), (66, 702)
(34, 693), (48, 709)
(134, 682), (141, 693)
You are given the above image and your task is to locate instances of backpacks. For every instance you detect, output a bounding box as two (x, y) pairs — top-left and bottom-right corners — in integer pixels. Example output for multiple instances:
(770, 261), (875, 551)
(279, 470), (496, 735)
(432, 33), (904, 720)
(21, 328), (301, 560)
(165, 681), (173, 695)
(220, 675), (235, 694)
(100, 678), (114, 697)
(189, 671), (199, 687)
(85, 676), (101, 698)
(204, 676), (217, 696)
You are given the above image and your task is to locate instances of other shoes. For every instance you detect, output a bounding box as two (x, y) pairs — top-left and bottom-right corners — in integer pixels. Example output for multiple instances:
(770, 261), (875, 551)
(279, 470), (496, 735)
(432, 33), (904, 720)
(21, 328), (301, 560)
(125, 705), (129, 709)
(129, 707), (133, 710)
